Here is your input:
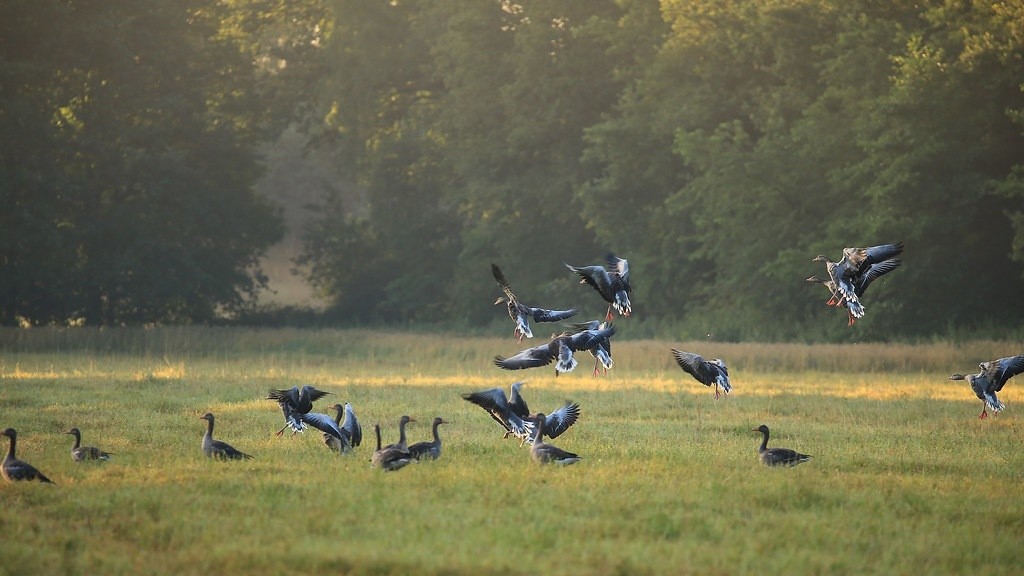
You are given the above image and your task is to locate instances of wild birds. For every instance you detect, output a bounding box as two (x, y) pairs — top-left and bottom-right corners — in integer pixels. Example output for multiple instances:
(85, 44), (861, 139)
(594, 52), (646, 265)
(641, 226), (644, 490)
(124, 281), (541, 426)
(752, 425), (813, 468)
(494, 319), (618, 378)
(670, 348), (731, 400)
(371, 416), (450, 472)
(563, 252), (633, 318)
(491, 262), (580, 344)
(265, 385), (362, 455)
(198, 412), (255, 461)
(65, 428), (116, 461)
(949, 355), (1024, 420)
(805, 241), (905, 327)
(0, 427), (56, 485)
(460, 382), (583, 467)
